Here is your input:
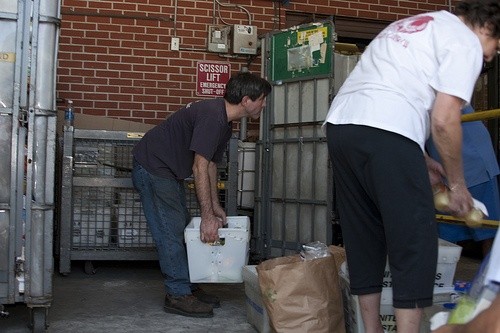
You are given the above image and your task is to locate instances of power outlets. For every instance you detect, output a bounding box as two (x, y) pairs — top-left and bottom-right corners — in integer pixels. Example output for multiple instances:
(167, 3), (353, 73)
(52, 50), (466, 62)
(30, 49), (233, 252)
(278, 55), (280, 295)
(170, 37), (180, 51)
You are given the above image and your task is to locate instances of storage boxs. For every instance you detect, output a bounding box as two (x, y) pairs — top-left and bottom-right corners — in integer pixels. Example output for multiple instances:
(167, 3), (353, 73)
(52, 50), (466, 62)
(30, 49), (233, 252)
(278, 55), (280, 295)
(434, 238), (463, 294)
(117, 205), (153, 248)
(73, 211), (110, 248)
(419, 296), (453, 333)
(184, 216), (251, 283)
(340, 274), (397, 333)
(242, 265), (271, 333)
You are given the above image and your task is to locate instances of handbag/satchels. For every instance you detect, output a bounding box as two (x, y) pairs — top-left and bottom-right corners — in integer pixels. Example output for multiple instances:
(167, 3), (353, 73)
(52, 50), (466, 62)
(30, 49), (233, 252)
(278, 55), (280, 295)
(256, 250), (345, 333)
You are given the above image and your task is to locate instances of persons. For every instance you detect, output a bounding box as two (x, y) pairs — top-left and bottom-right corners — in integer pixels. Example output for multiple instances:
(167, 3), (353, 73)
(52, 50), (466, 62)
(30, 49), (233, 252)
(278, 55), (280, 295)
(321, 0), (500, 333)
(131, 72), (271, 317)
(423, 103), (500, 262)
(428, 223), (500, 333)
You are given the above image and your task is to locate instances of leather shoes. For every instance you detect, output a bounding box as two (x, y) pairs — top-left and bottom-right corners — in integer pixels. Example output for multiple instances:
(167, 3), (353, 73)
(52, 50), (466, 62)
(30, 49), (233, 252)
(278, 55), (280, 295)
(191, 287), (220, 307)
(163, 293), (213, 317)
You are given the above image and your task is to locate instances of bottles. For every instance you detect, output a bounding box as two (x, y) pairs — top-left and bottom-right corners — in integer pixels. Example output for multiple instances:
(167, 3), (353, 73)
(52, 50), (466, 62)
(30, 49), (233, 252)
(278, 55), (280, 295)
(64, 100), (76, 129)
